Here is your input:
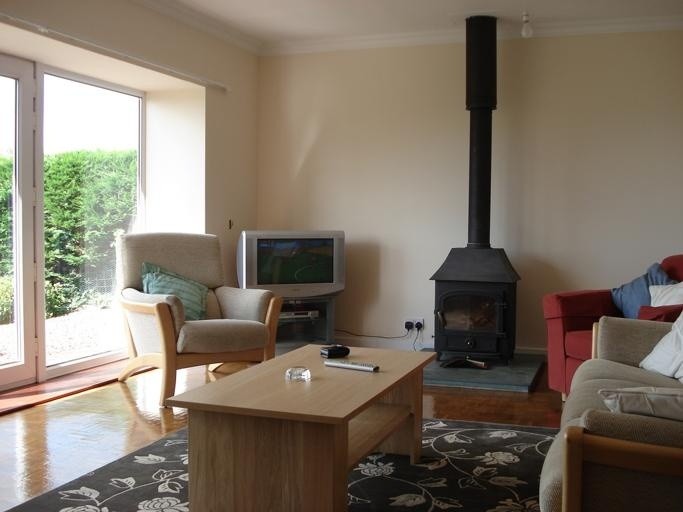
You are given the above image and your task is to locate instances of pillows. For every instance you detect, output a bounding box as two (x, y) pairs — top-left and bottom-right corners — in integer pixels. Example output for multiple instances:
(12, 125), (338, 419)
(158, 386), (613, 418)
(139, 260), (208, 321)
(598, 387), (683, 421)
(611, 262), (683, 381)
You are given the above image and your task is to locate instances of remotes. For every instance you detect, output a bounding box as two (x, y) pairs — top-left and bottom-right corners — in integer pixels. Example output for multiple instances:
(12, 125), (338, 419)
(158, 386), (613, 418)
(324, 359), (380, 372)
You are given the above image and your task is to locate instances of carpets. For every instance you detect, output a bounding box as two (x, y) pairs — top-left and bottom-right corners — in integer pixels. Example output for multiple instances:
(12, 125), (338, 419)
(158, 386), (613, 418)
(4, 415), (560, 512)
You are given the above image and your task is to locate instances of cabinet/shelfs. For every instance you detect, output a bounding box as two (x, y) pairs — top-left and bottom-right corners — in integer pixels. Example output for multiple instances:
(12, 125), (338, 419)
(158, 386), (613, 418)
(274, 296), (334, 357)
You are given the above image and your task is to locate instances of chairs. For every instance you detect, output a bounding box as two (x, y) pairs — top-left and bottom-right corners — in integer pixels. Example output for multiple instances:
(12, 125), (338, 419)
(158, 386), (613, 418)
(541, 254), (683, 401)
(115, 234), (282, 410)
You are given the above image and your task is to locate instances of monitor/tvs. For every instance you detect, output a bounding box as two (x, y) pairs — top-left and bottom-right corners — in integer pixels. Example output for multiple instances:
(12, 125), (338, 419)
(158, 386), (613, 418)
(236, 231), (345, 300)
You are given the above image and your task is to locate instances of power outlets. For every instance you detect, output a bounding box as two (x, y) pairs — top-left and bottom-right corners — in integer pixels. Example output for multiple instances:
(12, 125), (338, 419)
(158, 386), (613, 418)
(401, 319), (413, 339)
(413, 318), (424, 330)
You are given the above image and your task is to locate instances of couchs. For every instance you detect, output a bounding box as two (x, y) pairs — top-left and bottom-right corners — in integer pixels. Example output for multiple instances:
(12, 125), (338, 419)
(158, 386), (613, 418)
(539, 315), (683, 512)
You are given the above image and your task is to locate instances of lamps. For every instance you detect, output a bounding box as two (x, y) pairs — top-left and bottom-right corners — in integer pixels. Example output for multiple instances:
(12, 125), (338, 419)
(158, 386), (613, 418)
(521, 11), (534, 39)
